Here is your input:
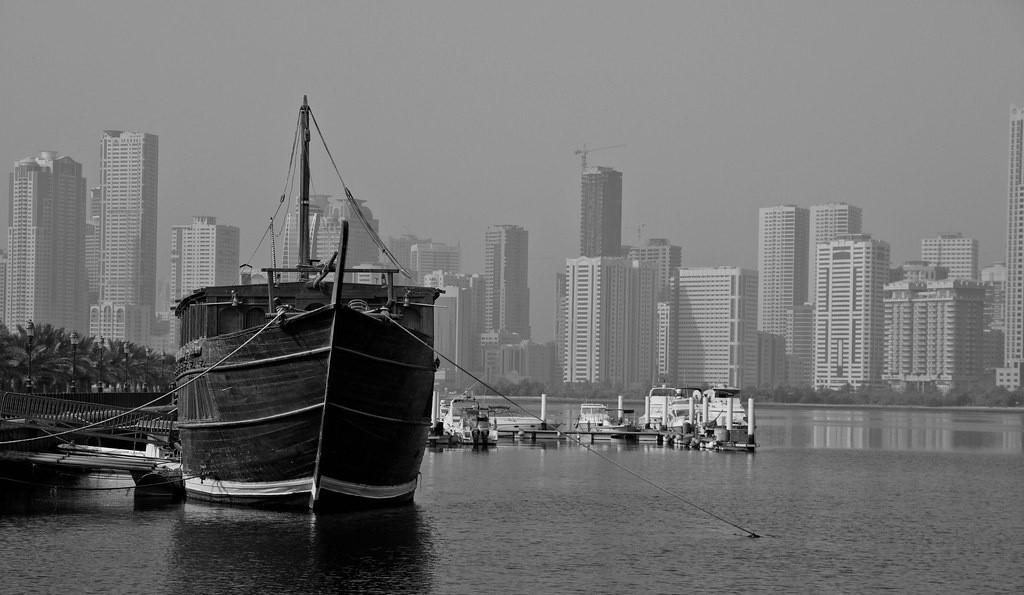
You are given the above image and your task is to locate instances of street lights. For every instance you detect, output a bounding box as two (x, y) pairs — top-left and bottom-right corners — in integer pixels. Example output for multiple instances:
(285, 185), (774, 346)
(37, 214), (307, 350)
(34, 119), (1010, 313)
(145, 346), (151, 393)
(69, 329), (80, 394)
(98, 336), (104, 392)
(123, 341), (130, 393)
(25, 318), (35, 393)
(161, 350), (166, 393)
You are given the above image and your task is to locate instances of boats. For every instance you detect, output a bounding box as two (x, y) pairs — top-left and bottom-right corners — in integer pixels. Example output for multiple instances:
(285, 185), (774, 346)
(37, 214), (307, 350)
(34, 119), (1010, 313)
(573, 397), (614, 429)
(488, 416), (561, 436)
(636, 369), (758, 432)
(440, 395), (499, 446)
(166, 93), (446, 516)
